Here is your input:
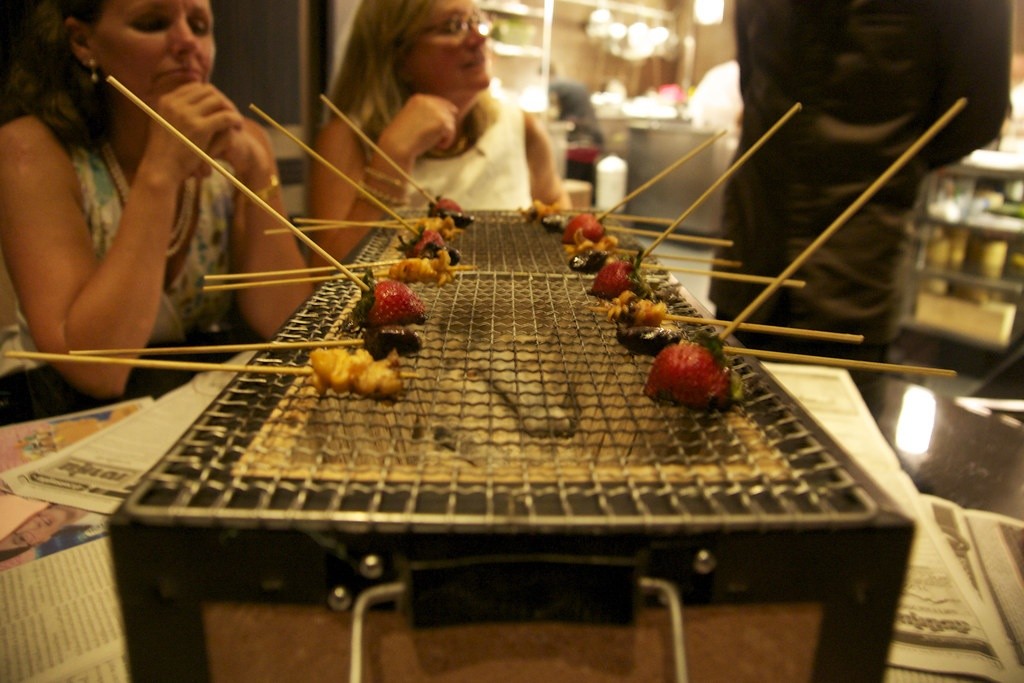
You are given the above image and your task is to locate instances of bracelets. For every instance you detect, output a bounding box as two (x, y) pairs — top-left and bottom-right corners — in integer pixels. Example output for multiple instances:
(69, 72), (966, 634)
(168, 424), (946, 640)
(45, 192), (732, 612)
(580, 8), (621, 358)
(240, 173), (282, 208)
(355, 178), (410, 206)
(364, 166), (410, 192)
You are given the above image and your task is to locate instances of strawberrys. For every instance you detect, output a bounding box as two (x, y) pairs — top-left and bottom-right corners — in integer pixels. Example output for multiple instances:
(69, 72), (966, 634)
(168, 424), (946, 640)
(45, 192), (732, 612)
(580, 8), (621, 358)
(556, 212), (603, 238)
(408, 225), (444, 254)
(593, 259), (638, 301)
(362, 277), (428, 317)
(643, 341), (727, 404)
(425, 195), (459, 215)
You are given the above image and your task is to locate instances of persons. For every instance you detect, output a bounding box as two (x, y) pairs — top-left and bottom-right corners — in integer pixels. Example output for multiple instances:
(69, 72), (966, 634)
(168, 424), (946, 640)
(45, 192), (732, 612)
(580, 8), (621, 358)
(307, 1), (562, 287)
(707, 0), (1014, 422)
(0, 0), (317, 401)
(538, 62), (604, 208)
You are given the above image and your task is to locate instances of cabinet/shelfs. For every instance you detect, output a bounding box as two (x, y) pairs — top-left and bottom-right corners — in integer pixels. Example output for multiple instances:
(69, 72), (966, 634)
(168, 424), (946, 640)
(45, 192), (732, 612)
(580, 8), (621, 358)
(897, 148), (1024, 358)
(475, 0), (555, 115)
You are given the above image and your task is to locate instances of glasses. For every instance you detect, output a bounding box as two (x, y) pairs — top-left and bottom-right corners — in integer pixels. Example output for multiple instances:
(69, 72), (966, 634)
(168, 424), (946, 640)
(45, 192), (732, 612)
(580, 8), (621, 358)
(417, 14), (494, 36)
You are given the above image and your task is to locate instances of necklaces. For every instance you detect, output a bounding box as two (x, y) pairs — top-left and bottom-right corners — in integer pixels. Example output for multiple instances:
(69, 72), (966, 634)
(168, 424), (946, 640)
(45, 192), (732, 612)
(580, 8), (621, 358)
(94, 132), (197, 258)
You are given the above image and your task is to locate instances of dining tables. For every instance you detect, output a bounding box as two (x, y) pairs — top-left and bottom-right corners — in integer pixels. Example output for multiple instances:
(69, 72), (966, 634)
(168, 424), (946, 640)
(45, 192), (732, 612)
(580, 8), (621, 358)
(0, 338), (1024, 683)
